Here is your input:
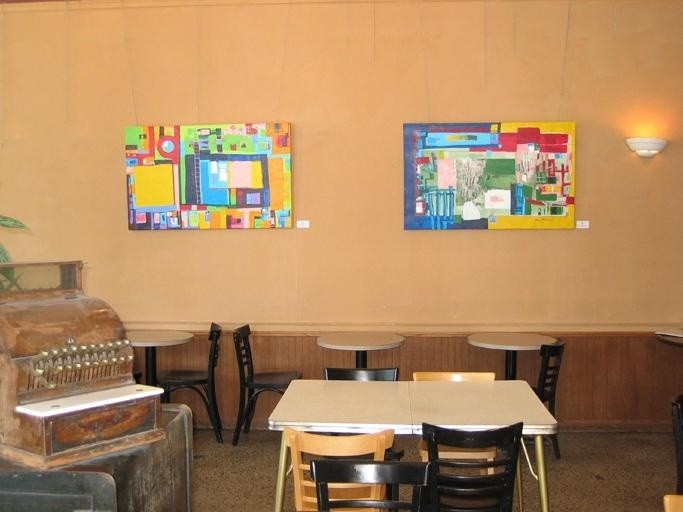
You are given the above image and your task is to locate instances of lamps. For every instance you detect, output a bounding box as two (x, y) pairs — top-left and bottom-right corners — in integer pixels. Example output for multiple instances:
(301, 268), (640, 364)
(624, 135), (667, 158)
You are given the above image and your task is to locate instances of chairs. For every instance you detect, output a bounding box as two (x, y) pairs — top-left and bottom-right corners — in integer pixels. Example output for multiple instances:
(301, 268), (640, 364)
(282, 424), (395, 511)
(420, 420), (524, 511)
(231, 324), (304, 449)
(529, 341), (568, 460)
(671, 390), (682, 494)
(308, 457), (434, 511)
(155, 320), (223, 442)
(323, 365), (405, 498)
(412, 369), (497, 476)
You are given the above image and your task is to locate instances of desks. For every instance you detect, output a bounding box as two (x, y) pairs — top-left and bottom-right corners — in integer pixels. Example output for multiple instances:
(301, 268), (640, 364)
(662, 494), (682, 512)
(266, 378), (561, 511)
(123, 329), (196, 386)
(653, 327), (683, 349)
(316, 330), (406, 369)
(464, 332), (558, 379)
